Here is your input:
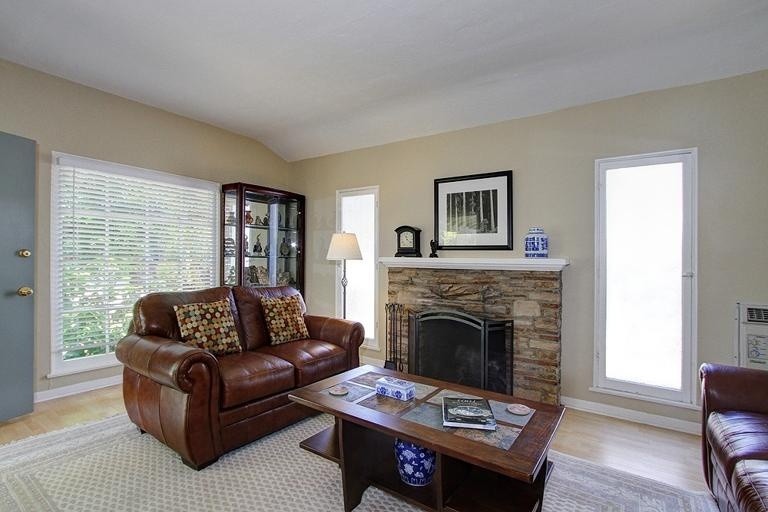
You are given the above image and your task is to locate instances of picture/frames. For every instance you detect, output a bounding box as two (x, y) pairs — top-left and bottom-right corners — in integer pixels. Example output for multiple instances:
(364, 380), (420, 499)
(433, 169), (514, 251)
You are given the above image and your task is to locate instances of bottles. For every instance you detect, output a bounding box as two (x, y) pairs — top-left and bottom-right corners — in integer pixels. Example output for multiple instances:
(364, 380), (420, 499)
(280, 237), (291, 257)
(245, 210), (254, 224)
(227, 211), (235, 223)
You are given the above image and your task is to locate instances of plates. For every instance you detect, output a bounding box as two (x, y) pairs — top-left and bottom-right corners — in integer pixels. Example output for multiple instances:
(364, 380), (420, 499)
(328, 385), (349, 396)
(505, 403), (531, 416)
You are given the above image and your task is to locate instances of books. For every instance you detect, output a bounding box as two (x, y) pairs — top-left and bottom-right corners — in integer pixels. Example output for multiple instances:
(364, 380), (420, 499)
(441, 395), (497, 433)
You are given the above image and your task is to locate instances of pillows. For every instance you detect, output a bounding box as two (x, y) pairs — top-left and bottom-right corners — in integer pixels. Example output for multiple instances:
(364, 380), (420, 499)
(261, 295), (310, 345)
(172, 297), (244, 355)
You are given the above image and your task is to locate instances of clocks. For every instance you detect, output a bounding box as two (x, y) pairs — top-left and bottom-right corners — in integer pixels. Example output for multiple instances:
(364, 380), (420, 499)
(393, 225), (423, 257)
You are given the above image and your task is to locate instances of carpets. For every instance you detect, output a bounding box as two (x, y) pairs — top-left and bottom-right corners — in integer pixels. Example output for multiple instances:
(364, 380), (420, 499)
(0, 412), (720, 511)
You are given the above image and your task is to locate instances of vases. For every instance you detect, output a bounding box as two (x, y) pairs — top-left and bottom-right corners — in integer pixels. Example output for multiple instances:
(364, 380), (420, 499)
(522, 226), (550, 258)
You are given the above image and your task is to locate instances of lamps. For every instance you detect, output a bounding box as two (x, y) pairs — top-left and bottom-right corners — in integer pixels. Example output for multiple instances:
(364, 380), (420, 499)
(325, 229), (363, 320)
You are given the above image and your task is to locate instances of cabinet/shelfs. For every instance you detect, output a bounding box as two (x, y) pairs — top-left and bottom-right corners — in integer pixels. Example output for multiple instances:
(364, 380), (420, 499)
(219, 182), (306, 294)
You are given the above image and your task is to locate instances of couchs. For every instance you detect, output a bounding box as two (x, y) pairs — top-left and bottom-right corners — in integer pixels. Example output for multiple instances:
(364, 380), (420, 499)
(115, 285), (365, 471)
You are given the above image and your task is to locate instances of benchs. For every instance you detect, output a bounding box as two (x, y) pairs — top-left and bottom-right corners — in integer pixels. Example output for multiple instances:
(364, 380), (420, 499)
(696, 361), (768, 510)
